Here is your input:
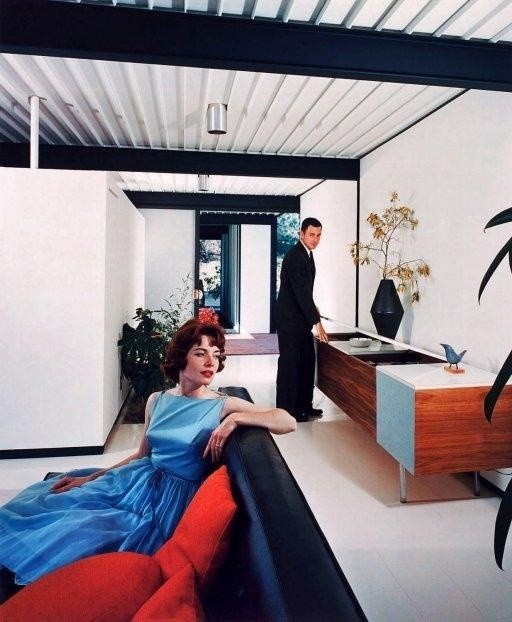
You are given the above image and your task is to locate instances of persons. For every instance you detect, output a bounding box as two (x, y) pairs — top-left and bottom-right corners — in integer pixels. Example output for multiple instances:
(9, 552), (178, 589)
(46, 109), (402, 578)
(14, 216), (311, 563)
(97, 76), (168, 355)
(276, 217), (328, 422)
(0, 321), (298, 601)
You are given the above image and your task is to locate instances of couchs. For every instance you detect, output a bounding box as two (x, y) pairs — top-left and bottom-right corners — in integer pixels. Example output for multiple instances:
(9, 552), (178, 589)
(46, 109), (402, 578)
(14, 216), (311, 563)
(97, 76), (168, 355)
(0, 383), (370, 622)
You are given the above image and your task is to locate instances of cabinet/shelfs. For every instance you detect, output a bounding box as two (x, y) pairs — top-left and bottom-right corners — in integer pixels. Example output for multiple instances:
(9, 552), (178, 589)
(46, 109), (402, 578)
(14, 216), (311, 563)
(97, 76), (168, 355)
(312, 324), (512, 504)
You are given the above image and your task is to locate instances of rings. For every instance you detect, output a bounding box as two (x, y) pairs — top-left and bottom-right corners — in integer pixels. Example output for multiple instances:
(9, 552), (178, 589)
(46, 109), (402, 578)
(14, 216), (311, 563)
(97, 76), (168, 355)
(215, 444), (219, 447)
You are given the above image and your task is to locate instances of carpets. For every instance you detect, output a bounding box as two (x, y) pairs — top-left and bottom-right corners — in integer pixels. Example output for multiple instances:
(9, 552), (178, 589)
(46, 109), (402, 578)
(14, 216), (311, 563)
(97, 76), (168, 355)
(121, 395), (147, 424)
(222, 332), (281, 355)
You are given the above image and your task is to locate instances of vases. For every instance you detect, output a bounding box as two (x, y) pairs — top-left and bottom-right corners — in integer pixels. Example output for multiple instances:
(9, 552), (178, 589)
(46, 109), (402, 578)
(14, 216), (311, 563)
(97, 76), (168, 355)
(349, 190), (430, 344)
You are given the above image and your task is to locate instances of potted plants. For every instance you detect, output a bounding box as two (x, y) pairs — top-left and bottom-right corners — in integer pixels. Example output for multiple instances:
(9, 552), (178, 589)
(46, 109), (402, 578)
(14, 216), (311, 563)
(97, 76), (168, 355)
(116, 272), (195, 398)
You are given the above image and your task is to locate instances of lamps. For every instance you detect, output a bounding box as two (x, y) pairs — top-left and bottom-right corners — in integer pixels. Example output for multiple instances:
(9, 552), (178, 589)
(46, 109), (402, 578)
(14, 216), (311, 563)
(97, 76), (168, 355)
(207, 102), (229, 135)
(197, 174), (209, 191)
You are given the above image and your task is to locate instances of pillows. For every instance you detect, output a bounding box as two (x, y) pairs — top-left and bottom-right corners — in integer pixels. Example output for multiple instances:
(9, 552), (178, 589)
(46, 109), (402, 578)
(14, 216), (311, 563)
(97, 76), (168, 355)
(0, 466), (237, 622)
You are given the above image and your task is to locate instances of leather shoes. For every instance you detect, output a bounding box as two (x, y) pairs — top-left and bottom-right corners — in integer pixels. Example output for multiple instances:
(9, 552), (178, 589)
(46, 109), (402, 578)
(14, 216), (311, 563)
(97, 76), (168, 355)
(292, 408), (308, 422)
(306, 405), (324, 416)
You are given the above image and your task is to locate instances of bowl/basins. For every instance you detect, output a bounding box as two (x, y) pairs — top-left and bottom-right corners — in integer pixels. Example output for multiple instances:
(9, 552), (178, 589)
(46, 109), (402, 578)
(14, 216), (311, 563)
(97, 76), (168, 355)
(349, 337), (373, 347)
